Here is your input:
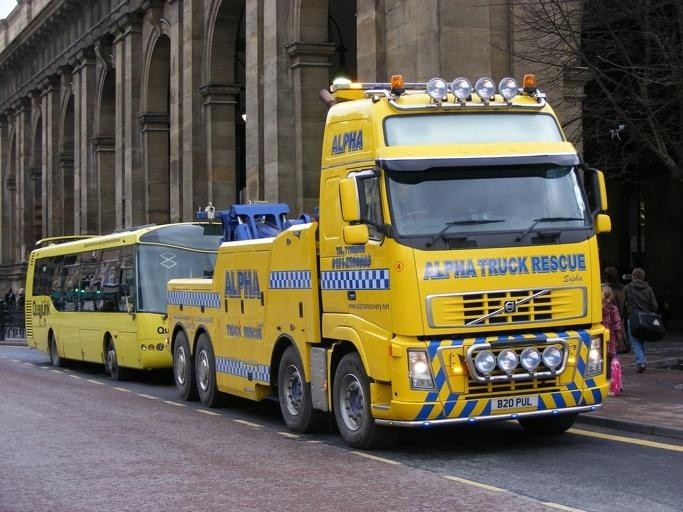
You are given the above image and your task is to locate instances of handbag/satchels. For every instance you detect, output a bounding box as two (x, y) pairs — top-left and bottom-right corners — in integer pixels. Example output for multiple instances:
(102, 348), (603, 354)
(630, 308), (666, 342)
(617, 317), (631, 354)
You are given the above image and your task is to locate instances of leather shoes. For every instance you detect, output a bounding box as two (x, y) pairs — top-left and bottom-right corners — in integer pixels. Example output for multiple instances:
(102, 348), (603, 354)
(637, 363), (645, 373)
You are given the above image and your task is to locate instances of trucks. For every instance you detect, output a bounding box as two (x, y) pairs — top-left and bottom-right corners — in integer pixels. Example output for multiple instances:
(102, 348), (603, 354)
(167, 74), (612, 449)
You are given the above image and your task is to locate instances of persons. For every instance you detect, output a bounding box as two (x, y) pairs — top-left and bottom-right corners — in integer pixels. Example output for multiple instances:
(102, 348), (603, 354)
(625, 267), (659, 373)
(603, 286), (621, 381)
(16, 287), (24, 306)
(601, 266), (630, 353)
(5, 289), (14, 305)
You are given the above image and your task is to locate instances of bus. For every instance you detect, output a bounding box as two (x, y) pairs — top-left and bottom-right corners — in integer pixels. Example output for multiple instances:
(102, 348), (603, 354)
(24, 222), (224, 380)
